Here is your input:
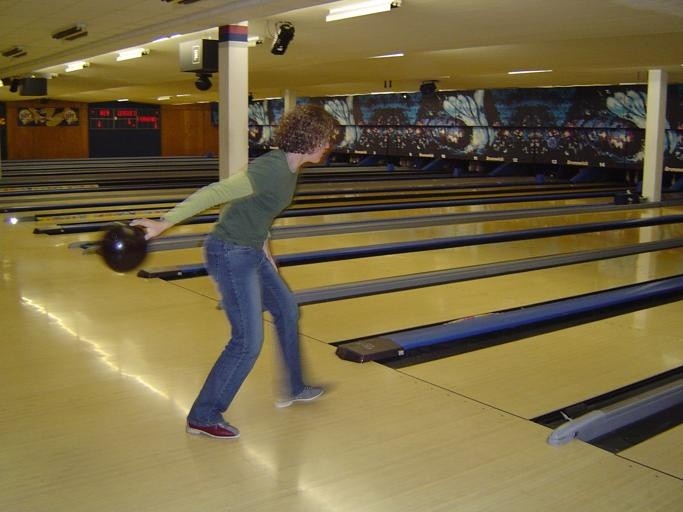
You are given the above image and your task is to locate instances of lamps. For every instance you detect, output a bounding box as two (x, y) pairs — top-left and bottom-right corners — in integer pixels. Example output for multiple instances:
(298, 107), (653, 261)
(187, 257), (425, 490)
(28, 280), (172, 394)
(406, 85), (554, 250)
(325, 0), (400, 22)
(65, 62), (90, 72)
(116, 48), (150, 61)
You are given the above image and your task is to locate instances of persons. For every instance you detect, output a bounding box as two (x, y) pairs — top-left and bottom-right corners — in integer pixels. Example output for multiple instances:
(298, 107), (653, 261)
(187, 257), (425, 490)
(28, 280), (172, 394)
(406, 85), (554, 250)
(128, 103), (337, 439)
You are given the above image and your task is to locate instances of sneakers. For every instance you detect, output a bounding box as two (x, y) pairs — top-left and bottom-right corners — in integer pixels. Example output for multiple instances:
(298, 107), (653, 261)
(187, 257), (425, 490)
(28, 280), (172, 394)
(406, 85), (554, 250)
(186, 420), (242, 439)
(273, 385), (325, 408)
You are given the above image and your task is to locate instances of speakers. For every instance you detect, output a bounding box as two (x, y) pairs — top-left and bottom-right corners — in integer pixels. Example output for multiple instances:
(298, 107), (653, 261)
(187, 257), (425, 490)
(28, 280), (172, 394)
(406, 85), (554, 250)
(19, 78), (48, 96)
(420, 83), (436, 95)
(179, 39), (219, 72)
